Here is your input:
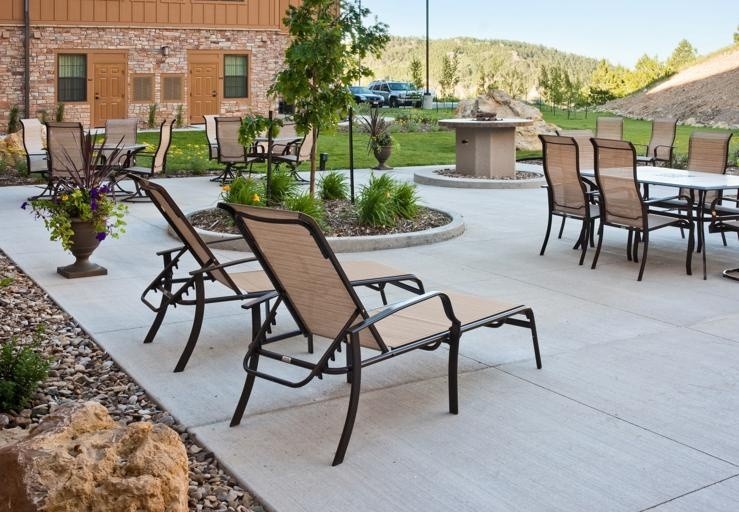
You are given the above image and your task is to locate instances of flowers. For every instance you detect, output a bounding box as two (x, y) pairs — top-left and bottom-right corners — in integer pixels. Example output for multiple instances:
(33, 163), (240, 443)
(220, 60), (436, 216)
(21, 128), (129, 251)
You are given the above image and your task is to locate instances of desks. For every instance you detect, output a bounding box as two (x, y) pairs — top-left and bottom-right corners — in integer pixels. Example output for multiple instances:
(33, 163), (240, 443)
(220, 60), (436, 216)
(90, 144), (149, 194)
(578, 165), (739, 256)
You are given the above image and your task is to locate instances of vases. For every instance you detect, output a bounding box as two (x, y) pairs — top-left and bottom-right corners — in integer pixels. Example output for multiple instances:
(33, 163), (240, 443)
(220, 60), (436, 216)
(56, 218), (108, 278)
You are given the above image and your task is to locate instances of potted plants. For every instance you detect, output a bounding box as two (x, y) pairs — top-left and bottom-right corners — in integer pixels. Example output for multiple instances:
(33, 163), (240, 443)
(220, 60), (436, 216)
(355, 104), (394, 170)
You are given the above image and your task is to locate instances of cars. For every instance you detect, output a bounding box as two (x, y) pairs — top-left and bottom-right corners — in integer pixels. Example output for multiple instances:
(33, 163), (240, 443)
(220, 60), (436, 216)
(347, 85), (385, 109)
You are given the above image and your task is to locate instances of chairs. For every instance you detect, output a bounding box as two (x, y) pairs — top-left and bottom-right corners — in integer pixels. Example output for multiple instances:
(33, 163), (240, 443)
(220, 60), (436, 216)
(44, 121), (100, 188)
(101, 118), (137, 195)
(708, 198), (739, 281)
(555, 129), (595, 205)
(121, 118), (178, 203)
(203, 114), (318, 185)
(538, 134), (602, 265)
(216, 202), (542, 467)
(588, 138), (695, 282)
(18, 118), (56, 198)
(595, 117), (624, 141)
(646, 132), (733, 253)
(125, 171), (425, 372)
(636, 118), (678, 171)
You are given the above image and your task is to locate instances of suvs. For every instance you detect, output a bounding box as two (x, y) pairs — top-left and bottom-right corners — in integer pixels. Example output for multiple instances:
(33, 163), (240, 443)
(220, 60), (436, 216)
(365, 79), (424, 109)
